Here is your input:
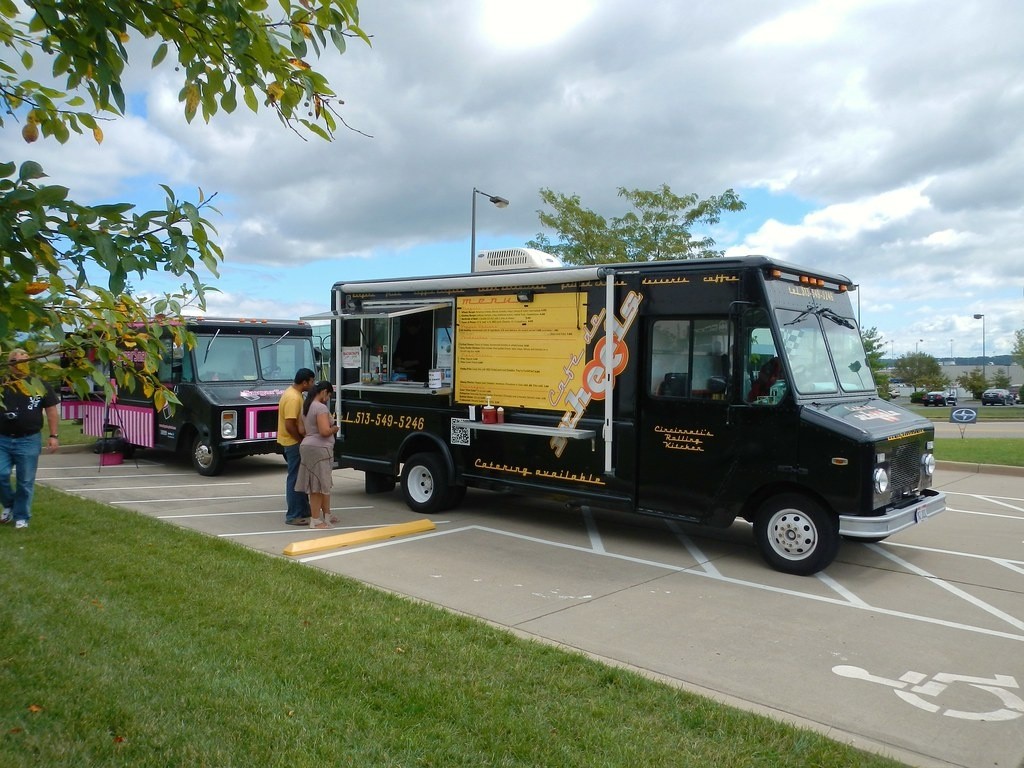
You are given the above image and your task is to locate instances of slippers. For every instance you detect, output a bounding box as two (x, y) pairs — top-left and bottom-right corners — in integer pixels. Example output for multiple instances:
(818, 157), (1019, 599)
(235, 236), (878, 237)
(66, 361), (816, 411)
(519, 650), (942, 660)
(286, 517), (307, 525)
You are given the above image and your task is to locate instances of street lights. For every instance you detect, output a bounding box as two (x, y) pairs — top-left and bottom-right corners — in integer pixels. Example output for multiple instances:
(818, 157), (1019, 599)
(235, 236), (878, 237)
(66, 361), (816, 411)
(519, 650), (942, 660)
(915, 338), (924, 354)
(974, 314), (985, 377)
(469, 186), (508, 275)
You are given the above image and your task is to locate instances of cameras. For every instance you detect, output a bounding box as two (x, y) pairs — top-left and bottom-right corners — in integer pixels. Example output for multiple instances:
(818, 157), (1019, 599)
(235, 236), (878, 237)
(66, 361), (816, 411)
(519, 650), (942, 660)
(4, 412), (18, 419)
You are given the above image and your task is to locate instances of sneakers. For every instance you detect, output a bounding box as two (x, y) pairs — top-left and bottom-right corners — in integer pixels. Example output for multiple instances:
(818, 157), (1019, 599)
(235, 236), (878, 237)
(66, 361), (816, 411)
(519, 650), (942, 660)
(0, 506), (13, 524)
(15, 520), (29, 529)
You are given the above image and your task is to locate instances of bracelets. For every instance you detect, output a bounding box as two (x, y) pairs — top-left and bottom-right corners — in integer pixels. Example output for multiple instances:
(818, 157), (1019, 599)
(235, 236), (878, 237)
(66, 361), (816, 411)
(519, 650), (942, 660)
(50, 434), (59, 437)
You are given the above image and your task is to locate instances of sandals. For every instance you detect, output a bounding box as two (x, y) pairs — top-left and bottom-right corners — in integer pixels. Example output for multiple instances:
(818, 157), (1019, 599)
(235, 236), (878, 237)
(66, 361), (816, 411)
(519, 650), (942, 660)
(323, 512), (340, 523)
(310, 516), (333, 529)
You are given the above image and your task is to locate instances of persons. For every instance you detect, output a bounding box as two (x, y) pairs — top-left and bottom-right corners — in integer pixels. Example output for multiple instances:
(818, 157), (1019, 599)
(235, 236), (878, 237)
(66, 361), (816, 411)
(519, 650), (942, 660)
(237, 351), (253, 371)
(748, 355), (784, 401)
(292, 380), (340, 529)
(0, 349), (60, 528)
(707, 342), (731, 377)
(276, 369), (315, 525)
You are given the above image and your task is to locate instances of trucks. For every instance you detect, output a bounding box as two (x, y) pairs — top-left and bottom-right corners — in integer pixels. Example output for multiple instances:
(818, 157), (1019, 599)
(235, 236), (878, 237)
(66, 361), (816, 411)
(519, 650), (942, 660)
(298, 254), (948, 577)
(61, 314), (324, 478)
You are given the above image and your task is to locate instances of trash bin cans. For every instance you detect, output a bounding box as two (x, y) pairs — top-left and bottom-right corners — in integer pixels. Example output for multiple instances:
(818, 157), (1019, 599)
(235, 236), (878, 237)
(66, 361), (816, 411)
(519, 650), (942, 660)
(100, 437), (123, 466)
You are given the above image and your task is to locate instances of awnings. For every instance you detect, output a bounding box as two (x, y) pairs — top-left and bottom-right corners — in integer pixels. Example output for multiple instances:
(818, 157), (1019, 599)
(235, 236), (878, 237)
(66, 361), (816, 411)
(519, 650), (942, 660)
(299, 301), (452, 342)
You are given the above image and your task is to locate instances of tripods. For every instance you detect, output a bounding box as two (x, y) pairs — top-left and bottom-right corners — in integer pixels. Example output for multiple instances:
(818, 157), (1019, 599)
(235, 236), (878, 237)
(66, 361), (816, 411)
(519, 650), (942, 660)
(98, 400), (138, 473)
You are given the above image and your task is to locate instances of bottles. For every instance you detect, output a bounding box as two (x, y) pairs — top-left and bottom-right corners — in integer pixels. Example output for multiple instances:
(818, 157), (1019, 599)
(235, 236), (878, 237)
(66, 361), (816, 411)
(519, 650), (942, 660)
(497, 406), (504, 423)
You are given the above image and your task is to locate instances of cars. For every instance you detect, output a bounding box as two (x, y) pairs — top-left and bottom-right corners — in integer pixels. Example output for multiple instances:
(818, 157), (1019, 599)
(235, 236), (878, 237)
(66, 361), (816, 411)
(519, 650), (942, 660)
(982, 388), (1015, 406)
(922, 390), (958, 407)
(887, 388), (901, 399)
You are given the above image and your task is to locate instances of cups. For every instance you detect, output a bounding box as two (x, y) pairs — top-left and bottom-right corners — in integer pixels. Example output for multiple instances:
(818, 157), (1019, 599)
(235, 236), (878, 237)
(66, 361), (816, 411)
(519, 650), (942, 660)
(469, 404), (482, 422)
